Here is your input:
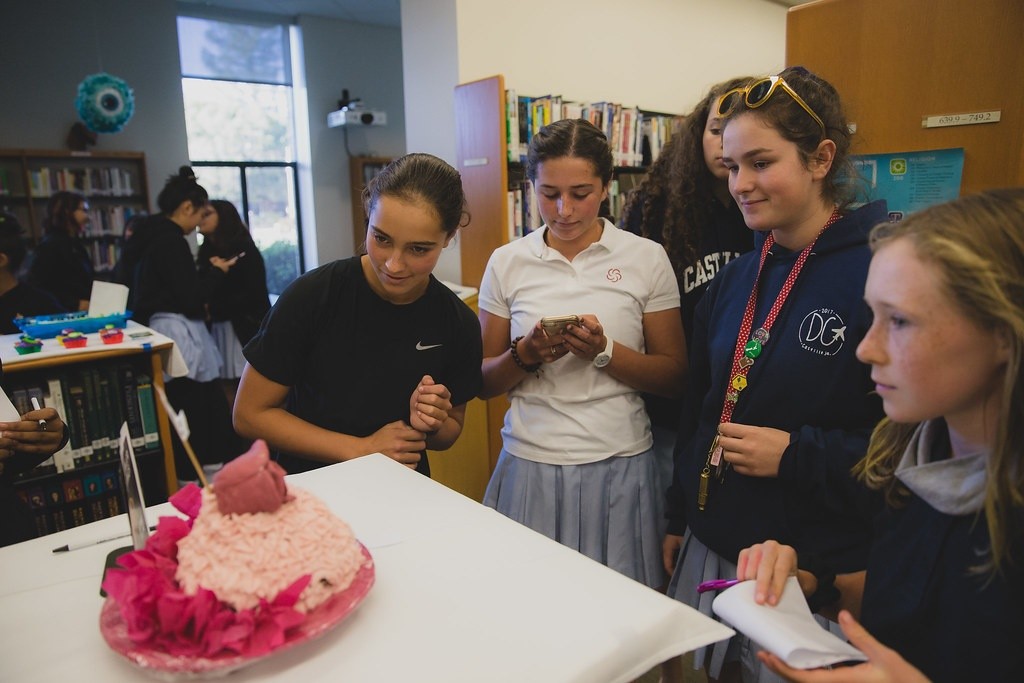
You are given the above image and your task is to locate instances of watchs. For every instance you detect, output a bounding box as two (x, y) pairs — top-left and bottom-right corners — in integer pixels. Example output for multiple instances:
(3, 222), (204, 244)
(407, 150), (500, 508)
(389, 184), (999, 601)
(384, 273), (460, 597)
(593, 338), (614, 368)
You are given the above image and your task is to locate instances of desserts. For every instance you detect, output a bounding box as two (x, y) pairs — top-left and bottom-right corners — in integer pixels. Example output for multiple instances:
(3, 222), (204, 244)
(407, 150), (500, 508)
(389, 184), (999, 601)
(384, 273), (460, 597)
(56, 328), (87, 348)
(175, 441), (363, 614)
(98, 324), (123, 344)
(14, 335), (44, 354)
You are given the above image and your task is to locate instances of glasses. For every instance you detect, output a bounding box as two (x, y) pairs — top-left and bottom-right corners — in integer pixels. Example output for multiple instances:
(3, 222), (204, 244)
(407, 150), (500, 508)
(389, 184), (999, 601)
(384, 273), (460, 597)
(202, 210), (217, 218)
(77, 207), (89, 214)
(717, 76), (825, 143)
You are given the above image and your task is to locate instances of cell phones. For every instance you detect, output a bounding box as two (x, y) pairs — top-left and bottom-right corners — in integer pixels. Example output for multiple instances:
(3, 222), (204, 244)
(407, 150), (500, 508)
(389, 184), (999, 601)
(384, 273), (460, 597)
(225, 250), (245, 262)
(541, 315), (581, 349)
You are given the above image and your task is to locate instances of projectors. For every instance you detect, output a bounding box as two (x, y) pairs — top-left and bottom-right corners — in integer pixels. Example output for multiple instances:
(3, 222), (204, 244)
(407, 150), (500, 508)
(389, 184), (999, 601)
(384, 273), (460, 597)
(327, 110), (386, 128)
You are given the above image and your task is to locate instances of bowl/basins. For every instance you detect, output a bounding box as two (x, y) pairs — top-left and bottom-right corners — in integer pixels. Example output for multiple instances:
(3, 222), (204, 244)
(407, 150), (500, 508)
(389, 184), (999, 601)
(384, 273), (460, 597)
(14, 329), (123, 355)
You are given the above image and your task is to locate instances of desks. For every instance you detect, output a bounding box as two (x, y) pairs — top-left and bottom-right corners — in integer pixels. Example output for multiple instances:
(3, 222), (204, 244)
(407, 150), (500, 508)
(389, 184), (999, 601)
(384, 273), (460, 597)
(1, 453), (736, 682)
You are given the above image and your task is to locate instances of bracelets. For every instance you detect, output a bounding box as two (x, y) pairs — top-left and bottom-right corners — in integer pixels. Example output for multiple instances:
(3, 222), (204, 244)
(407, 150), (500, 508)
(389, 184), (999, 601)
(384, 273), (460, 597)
(799, 553), (836, 612)
(511, 335), (544, 379)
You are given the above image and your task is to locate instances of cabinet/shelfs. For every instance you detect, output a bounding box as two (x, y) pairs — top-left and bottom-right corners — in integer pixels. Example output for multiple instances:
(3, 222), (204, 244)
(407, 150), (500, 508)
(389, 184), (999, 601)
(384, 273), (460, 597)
(0, 148), (153, 283)
(452, 75), (689, 477)
(1, 320), (190, 546)
(350, 156), (396, 255)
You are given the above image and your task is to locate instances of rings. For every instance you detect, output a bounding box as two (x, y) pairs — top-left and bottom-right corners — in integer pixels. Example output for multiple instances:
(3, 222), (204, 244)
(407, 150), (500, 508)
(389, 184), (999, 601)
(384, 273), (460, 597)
(551, 346), (557, 356)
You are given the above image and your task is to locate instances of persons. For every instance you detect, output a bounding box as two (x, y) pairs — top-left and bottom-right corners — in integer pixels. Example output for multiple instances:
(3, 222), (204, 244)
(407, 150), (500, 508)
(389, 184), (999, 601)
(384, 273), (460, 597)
(478, 118), (688, 590)
(231, 153), (484, 478)
(114, 164), (236, 480)
(197, 198), (271, 463)
(619, 76), (764, 516)
(26, 190), (94, 317)
(662, 66), (884, 682)
(0, 217), (33, 335)
(737, 190), (1024, 682)
(0, 360), (70, 546)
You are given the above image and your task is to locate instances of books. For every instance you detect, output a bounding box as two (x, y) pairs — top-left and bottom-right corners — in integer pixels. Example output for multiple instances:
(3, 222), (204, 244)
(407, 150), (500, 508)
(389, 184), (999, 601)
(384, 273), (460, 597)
(508, 178), (545, 241)
(0, 162), (147, 270)
(443, 280), (478, 300)
(365, 164), (388, 184)
(607, 180), (629, 225)
(506, 89), (684, 167)
(0, 366), (169, 536)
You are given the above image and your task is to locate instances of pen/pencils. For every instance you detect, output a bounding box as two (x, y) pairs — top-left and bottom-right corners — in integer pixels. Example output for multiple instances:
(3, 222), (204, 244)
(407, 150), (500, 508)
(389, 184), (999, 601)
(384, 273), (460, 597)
(31, 396), (48, 431)
(52, 524), (158, 554)
(693, 580), (741, 591)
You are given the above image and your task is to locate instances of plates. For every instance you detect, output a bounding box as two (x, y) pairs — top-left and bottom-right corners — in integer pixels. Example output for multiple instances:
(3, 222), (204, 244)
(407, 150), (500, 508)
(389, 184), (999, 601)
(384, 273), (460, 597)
(99, 539), (374, 671)
